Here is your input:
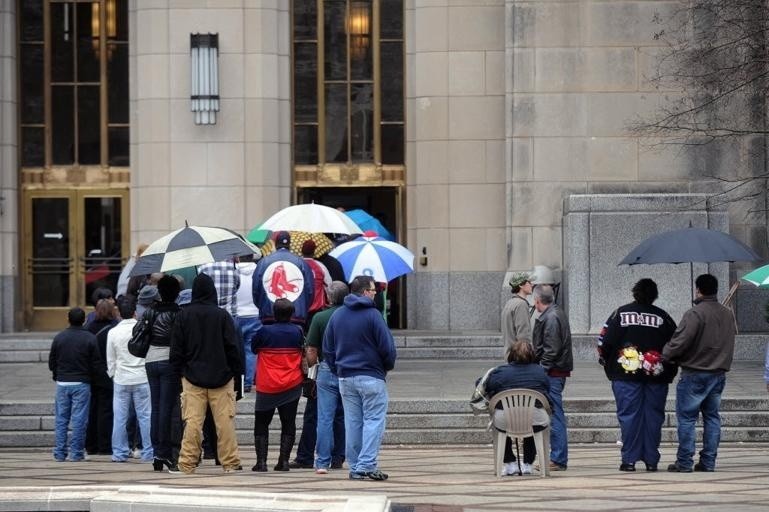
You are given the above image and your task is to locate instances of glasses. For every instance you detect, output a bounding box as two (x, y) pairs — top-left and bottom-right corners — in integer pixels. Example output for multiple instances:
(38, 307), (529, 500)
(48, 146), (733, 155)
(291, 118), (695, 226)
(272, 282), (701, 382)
(366, 287), (379, 293)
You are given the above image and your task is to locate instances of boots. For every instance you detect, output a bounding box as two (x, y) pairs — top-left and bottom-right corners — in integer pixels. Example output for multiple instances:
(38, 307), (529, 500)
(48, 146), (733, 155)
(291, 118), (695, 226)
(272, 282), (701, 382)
(274, 434), (295, 471)
(252, 435), (268, 472)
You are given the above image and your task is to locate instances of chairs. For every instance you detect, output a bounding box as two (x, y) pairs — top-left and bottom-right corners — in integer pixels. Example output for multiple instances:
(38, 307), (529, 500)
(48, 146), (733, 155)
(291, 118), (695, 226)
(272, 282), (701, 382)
(719, 281), (741, 336)
(488, 387), (552, 477)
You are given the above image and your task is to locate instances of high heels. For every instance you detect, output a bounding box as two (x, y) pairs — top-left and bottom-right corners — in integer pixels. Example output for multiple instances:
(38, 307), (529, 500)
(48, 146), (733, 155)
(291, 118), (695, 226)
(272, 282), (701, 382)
(154, 458), (176, 471)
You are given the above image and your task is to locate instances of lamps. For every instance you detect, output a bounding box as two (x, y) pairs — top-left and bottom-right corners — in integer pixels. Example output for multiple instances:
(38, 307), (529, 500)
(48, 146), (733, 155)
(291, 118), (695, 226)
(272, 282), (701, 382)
(190, 32), (219, 124)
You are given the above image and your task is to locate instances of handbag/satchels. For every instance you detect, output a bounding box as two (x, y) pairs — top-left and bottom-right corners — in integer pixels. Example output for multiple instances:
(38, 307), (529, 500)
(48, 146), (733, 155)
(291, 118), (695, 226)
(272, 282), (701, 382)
(169, 312), (186, 367)
(295, 324), (309, 374)
(128, 311), (153, 358)
(302, 378), (317, 400)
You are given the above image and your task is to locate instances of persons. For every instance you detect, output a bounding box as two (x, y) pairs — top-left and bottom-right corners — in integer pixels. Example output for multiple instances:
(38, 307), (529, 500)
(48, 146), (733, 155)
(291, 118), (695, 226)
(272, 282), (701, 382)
(82, 287), (115, 329)
(171, 274), (193, 306)
(167, 272), (243, 473)
(107, 295), (154, 463)
(112, 305), (147, 459)
(48, 308), (97, 462)
(660, 274), (735, 472)
(251, 298), (304, 472)
(234, 253), (263, 392)
(531, 283), (573, 470)
(197, 259), (242, 401)
(501, 272), (537, 461)
(132, 275), (185, 473)
(321, 276), (396, 481)
(252, 231), (314, 334)
(598, 278), (677, 471)
(84, 299), (117, 462)
(112, 241), (150, 299)
(301, 239), (333, 333)
(475, 335), (551, 476)
(289, 281), (347, 473)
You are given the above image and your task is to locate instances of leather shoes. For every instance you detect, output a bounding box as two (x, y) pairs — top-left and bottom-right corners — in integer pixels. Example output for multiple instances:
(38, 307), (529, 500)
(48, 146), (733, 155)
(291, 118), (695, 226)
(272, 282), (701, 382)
(331, 459), (342, 469)
(646, 464), (658, 471)
(288, 461), (313, 468)
(620, 462), (636, 471)
(534, 463), (566, 472)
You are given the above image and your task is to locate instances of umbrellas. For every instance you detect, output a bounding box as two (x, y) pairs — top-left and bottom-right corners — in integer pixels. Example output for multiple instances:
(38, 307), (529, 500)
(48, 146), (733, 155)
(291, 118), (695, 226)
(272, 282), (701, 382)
(260, 231), (336, 258)
(742, 264), (769, 288)
(257, 199), (364, 241)
(327, 236), (415, 284)
(617, 221), (764, 307)
(244, 222), (272, 246)
(124, 217), (261, 279)
(342, 209), (395, 243)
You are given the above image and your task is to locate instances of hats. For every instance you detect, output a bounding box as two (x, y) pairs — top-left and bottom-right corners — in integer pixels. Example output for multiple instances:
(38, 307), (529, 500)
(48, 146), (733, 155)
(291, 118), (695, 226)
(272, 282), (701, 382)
(275, 231), (291, 249)
(178, 289), (192, 306)
(303, 240), (319, 250)
(137, 285), (161, 305)
(509, 272), (536, 287)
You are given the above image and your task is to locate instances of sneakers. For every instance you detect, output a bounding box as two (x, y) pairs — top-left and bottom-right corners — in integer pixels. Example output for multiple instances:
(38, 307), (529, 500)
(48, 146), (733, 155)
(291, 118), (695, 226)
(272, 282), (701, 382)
(694, 463), (714, 472)
(130, 447), (142, 459)
(111, 457), (126, 462)
(135, 458), (153, 464)
(349, 472), (369, 480)
(500, 462), (520, 477)
(69, 457), (85, 462)
(168, 466), (185, 475)
(224, 466), (243, 474)
(84, 454), (114, 462)
(522, 462), (533, 474)
(128, 448), (134, 458)
(316, 468), (328, 474)
(355, 465), (388, 480)
(54, 459), (66, 461)
(668, 464), (693, 472)
